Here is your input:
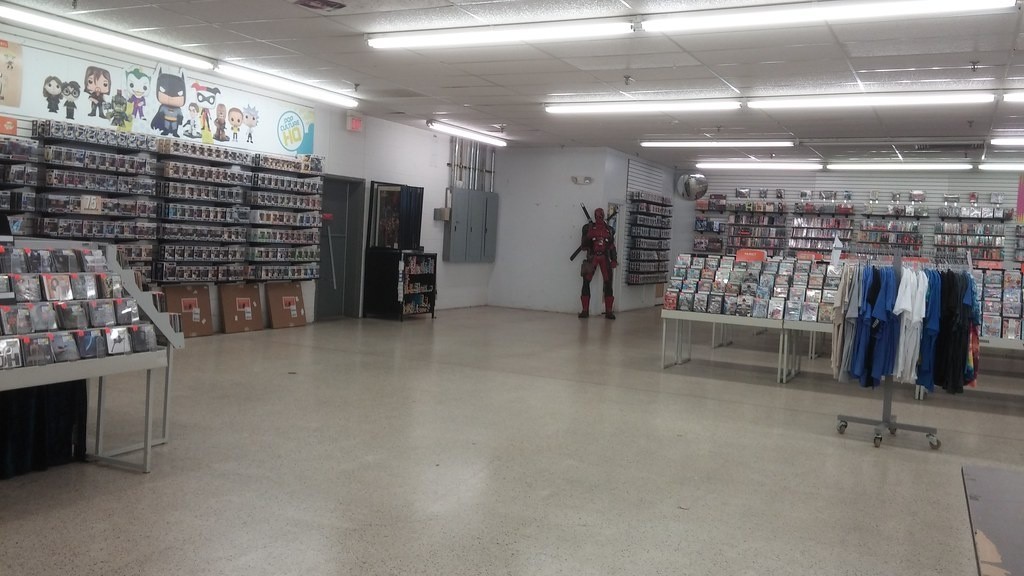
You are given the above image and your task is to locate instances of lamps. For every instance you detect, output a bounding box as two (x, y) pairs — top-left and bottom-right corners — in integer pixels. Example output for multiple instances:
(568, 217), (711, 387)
(639, 0), (1024, 33)
(989, 129), (1024, 145)
(695, 158), (826, 170)
(0, 0), (217, 70)
(542, 87), (746, 113)
(826, 157), (976, 170)
(212, 60), (361, 109)
(426, 119), (510, 148)
(746, 79), (1000, 109)
(975, 158), (1024, 171)
(1001, 79), (1024, 101)
(638, 139), (797, 148)
(364, 15), (639, 50)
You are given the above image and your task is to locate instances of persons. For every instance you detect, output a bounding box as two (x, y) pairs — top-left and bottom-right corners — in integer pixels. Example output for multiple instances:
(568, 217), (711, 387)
(577, 208), (618, 319)
(49, 277), (65, 301)
(13, 276), (31, 301)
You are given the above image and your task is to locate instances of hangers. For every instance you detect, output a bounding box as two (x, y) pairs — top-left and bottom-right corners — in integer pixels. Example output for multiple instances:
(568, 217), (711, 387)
(845, 244), (969, 276)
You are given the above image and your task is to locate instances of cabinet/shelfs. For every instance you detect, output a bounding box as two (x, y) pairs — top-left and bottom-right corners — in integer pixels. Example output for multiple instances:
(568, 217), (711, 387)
(363, 248), (438, 323)
(660, 252), (797, 383)
(919, 265), (1024, 400)
(0, 236), (186, 482)
(782, 260), (921, 399)
